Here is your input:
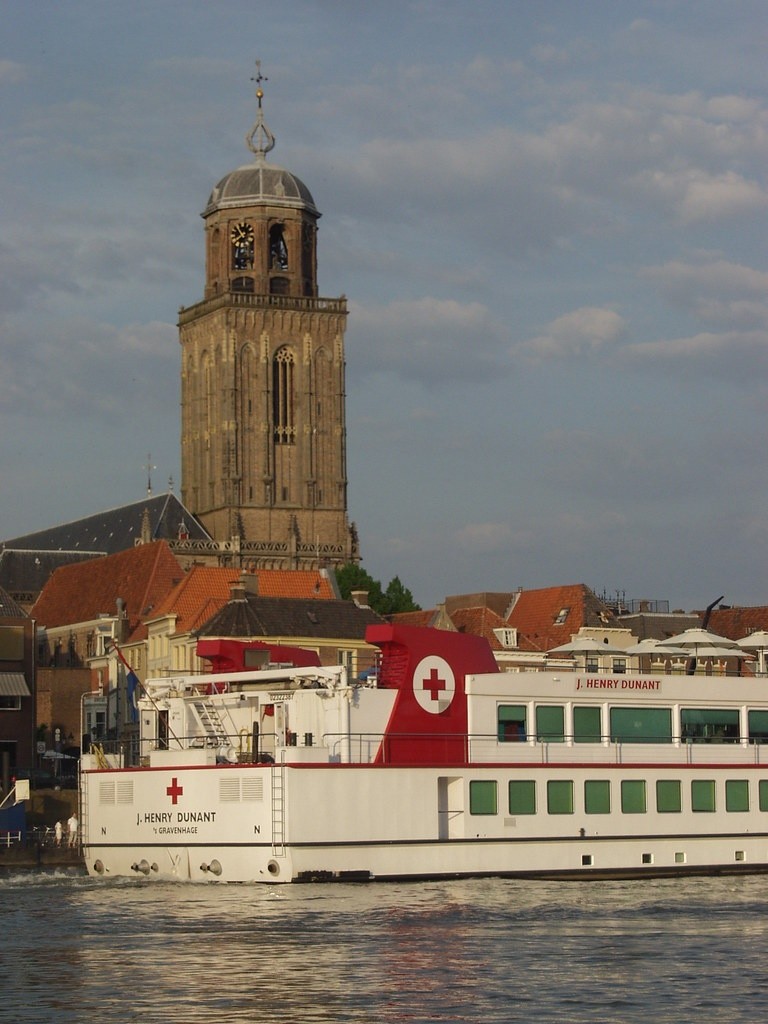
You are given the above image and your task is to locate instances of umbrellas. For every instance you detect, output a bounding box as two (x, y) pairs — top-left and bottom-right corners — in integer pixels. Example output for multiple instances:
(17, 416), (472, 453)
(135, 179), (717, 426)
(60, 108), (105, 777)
(547, 626), (768, 676)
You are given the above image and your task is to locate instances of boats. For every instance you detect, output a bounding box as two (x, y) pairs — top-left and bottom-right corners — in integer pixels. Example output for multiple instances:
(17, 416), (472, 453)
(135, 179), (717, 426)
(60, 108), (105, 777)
(78, 623), (768, 886)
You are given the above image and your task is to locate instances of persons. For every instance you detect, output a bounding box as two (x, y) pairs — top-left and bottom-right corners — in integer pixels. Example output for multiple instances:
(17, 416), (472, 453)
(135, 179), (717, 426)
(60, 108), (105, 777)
(55, 818), (66, 846)
(67, 812), (78, 847)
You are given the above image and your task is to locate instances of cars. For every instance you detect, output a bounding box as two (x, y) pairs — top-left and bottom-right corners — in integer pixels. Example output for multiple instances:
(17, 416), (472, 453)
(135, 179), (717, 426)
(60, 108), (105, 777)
(9, 767), (60, 791)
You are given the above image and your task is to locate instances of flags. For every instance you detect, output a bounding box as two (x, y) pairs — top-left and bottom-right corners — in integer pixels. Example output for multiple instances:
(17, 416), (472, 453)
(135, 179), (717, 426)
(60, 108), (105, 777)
(116, 648), (139, 723)
